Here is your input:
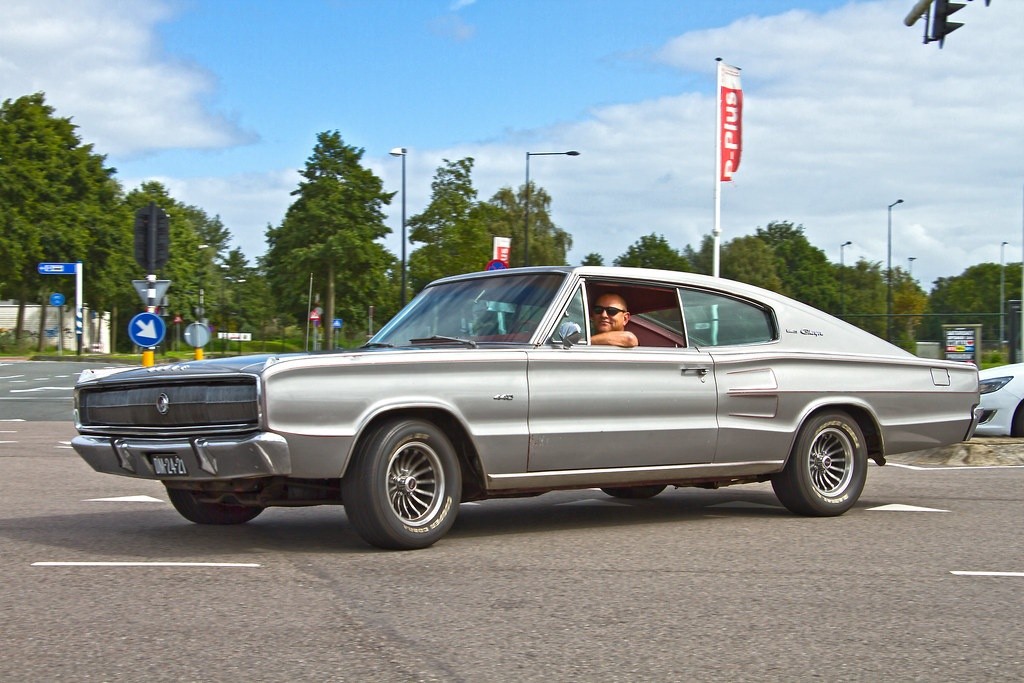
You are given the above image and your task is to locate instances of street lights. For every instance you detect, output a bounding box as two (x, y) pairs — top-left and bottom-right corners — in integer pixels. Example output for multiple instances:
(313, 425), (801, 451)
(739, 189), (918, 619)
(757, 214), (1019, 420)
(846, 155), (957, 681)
(886, 198), (904, 342)
(1000, 241), (1010, 350)
(389, 147), (406, 309)
(524, 151), (581, 265)
(196, 244), (246, 353)
(907, 256), (916, 279)
(841, 240), (852, 313)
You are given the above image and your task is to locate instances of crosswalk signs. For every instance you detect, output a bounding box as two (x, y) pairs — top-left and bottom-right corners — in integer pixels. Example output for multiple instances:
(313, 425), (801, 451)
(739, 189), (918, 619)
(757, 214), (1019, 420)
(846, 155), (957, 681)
(332, 319), (342, 329)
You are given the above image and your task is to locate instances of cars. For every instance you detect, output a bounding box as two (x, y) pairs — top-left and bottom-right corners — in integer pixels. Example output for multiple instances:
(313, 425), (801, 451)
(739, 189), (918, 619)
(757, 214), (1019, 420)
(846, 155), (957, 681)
(69, 264), (985, 550)
(975, 362), (1024, 438)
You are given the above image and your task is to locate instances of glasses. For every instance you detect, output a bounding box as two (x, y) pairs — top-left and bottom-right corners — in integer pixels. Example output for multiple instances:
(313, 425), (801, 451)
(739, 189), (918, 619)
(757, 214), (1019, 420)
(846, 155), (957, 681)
(590, 305), (625, 316)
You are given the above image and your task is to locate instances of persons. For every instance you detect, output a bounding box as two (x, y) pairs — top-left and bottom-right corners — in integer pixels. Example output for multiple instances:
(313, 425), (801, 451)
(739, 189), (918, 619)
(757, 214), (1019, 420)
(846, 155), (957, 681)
(590, 291), (639, 347)
